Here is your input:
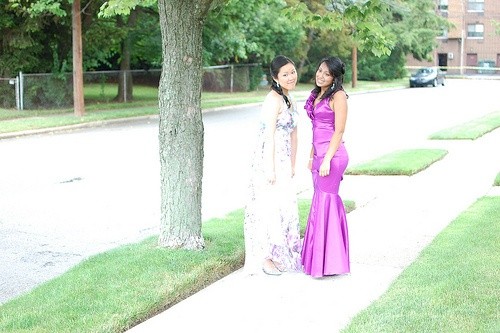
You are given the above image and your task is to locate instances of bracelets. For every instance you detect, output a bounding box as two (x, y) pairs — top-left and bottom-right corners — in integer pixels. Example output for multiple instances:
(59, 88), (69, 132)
(309, 158), (313, 160)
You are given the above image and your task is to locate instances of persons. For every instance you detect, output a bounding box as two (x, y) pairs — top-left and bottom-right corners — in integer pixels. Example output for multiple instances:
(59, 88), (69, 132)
(300, 56), (350, 278)
(244, 56), (301, 276)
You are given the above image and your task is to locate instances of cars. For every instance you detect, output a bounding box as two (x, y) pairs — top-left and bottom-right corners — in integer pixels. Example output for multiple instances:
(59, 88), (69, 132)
(409, 67), (445, 88)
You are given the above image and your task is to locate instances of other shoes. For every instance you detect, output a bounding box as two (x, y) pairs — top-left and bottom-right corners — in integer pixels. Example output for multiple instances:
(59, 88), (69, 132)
(263, 260), (282, 275)
(273, 261), (288, 272)
